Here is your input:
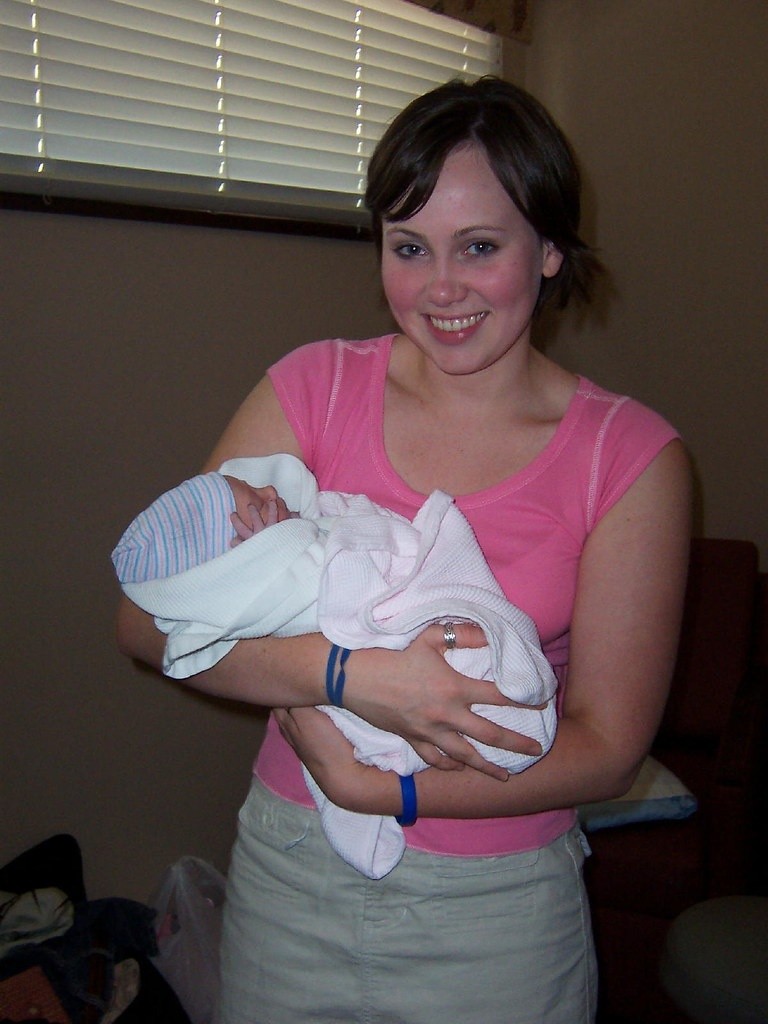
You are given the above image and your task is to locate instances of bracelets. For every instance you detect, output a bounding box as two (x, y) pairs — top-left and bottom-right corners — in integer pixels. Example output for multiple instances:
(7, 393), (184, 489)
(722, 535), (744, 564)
(326, 643), (352, 707)
(395, 771), (417, 827)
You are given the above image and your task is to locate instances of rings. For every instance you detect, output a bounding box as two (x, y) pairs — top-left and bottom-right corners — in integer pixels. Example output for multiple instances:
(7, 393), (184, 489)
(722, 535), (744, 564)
(442, 623), (456, 648)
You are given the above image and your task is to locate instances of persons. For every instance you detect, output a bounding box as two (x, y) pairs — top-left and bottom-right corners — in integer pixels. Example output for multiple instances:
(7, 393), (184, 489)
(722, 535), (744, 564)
(115, 73), (692, 1024)
(111, 454), (560, 880)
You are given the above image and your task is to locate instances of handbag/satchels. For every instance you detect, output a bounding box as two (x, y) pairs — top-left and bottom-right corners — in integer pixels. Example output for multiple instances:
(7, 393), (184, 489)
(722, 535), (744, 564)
(146, 853), (229, 1024)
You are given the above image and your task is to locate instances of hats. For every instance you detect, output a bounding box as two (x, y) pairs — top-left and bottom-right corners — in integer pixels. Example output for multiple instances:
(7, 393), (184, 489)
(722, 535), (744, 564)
(111, 470), (236, 583)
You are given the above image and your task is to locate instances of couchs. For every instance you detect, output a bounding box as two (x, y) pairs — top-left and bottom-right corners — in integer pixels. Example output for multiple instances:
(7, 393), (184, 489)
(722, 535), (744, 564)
(584, 536), (767, 1024)
